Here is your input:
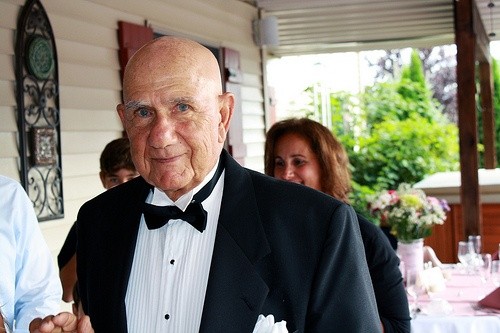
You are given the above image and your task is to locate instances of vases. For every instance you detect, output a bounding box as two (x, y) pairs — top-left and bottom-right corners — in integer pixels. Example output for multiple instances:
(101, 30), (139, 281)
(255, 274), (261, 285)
(397, 239), (425, 284)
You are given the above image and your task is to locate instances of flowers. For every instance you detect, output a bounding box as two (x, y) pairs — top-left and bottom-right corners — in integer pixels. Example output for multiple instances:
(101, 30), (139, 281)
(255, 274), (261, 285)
(369, 184), (451, 238)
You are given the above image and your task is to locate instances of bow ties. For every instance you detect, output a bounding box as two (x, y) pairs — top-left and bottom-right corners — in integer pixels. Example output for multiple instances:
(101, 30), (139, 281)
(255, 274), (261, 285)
(142, 155), (225, 233)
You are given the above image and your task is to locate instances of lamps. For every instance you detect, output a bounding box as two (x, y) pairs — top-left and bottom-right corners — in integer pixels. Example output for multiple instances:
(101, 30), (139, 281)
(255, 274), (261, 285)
(251, 15), (281, 48)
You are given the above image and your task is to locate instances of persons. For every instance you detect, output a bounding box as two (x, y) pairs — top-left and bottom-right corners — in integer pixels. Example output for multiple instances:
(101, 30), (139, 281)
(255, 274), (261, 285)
(58, 138), (142, 302)
(27, 35), (384, 333)
(0, 173), (74, 333)
(263, 117), (412, 332)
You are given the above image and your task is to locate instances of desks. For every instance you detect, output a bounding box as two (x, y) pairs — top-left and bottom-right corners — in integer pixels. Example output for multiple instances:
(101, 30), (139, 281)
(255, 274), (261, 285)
(406, 262), (500, 333)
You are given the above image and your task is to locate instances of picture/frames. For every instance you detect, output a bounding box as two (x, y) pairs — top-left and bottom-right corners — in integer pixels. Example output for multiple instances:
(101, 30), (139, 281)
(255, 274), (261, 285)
(30, 127), (57, 165)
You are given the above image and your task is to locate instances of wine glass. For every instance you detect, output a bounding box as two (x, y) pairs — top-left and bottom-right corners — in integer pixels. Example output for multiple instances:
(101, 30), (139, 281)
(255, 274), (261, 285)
(406, 268), (422, 315)
(458, 236), (492, 288)
